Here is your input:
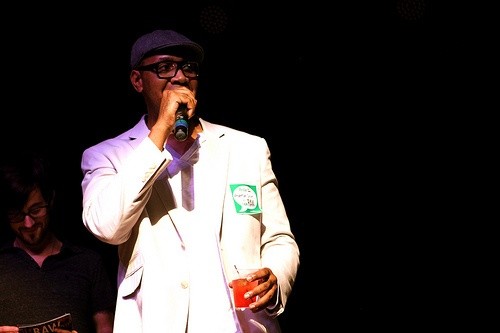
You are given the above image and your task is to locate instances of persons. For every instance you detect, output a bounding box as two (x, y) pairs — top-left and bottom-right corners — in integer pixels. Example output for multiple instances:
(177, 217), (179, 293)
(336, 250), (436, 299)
(80, 30), (300, 333)
(0, 169), (117, 333)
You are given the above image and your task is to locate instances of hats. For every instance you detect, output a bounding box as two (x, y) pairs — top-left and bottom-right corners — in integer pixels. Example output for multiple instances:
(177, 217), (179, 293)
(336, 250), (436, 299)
(130, 30), (204, 70)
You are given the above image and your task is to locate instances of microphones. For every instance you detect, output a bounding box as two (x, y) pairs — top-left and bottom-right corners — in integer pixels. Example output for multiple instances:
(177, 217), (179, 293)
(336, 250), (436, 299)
(175, 104), (189, 142)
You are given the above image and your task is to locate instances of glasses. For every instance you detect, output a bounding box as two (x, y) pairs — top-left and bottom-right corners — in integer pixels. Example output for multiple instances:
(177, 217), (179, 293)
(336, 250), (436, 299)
(8, 202), (49, 224)
(136, 61), (199, 79)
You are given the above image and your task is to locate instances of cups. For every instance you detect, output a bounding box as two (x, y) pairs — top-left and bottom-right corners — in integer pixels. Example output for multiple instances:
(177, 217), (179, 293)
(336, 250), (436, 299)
(232, 269), (258, 308)
